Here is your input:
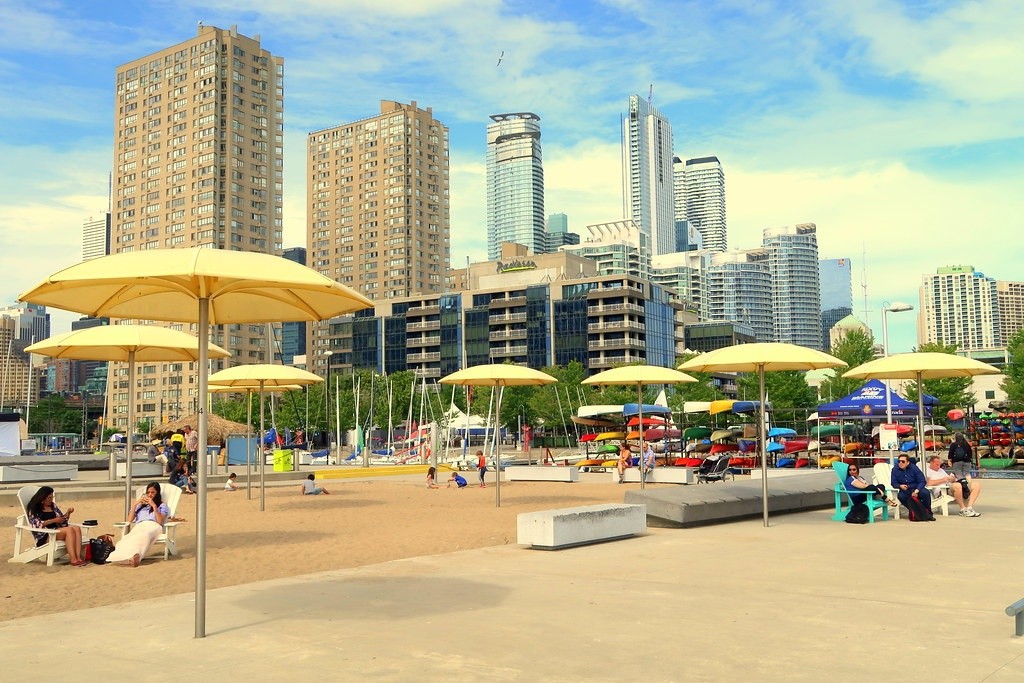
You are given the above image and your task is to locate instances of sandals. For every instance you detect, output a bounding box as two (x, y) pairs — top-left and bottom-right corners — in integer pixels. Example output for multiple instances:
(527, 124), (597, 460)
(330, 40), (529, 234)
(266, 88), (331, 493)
(887, 499), (897, 507)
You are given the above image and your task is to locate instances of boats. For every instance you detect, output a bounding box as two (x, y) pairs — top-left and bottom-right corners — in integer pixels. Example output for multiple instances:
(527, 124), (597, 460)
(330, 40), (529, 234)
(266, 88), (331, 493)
(537, 396), (1024, 467)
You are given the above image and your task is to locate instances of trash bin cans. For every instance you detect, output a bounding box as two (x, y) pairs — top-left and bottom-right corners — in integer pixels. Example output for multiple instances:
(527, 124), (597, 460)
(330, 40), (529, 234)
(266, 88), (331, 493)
(273, 450), (293, 471)
(533, 435), (576, 447)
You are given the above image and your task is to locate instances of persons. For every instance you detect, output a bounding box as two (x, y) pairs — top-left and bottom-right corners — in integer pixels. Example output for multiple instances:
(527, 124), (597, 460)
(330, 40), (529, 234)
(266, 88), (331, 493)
(701, 436), (711, 445)
(930, 455), (982, 518)
(475, 450), (486, 488)
(448, 472), (468, 488)
(637, 443), (655, 484)
(27, 486), (88, 567)
(147, 425), (198, 493)
(616, 443), (633, 485)
(302, 474), (329, 494)
(891, 455), (937, 522)
(948, 432), (973, 491)
(104, 480), (170, 567)
(843, 463), (898, 508)
(224, 472), (239, 492)
(426, 466), (451, 489)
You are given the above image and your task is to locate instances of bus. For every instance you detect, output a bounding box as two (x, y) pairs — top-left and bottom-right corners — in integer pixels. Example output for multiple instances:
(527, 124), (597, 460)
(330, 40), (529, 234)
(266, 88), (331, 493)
(27, 433), (80, 449)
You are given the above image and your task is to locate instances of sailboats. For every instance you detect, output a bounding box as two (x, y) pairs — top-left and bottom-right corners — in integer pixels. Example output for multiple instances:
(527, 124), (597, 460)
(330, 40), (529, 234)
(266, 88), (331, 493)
(311, 365), (536, 464)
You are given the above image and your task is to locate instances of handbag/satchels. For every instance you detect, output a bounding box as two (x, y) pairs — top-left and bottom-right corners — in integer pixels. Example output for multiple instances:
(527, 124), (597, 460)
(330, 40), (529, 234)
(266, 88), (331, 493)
(90, 538), (111, 564)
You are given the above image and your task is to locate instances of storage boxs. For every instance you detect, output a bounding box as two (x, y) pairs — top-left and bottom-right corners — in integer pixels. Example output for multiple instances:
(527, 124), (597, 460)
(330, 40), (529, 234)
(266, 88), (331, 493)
(206, 445), (221, 454)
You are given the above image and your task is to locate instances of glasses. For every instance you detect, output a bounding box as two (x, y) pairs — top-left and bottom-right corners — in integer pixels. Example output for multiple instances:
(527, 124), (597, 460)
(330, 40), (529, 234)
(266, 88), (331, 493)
(848, 469), (856, 472)
(644, 447), (647, 448)
(898, 460), (907, 463)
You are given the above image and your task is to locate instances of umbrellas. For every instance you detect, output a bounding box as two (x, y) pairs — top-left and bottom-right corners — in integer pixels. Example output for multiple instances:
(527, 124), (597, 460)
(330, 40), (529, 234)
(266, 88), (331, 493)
(678, 338), (846, 528)
(438, 365), (558, 506)
(23, 324), (230, 536)
(109, 433), (123, 443)
(16, 246), (376, 638)
(844, 351), (1002, 479)
(580, 365), (699, 490)
(204, 362), (324, 513)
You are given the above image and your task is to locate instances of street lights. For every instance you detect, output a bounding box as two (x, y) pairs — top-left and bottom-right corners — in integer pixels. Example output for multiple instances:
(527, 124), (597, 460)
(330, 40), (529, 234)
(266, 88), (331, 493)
(517, 405), (526, 453)
(882, 298), (915, 464)
(324, 350), (334, 465)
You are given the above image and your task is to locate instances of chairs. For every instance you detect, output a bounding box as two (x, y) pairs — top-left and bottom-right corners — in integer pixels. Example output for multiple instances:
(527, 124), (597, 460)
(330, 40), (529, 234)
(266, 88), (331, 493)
(832, 461), (891, 523)
(113, 482), (187, 561)
(7, 485), (99, 566)
(872, 462), (909, 519)
(917, 461), (955, 515)
(697, 455), (734, 484)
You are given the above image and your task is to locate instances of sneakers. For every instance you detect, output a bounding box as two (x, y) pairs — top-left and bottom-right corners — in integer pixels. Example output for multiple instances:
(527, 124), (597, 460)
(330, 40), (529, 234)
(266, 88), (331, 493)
(969, 508), (981, 517)
(959, 509), (976, 517)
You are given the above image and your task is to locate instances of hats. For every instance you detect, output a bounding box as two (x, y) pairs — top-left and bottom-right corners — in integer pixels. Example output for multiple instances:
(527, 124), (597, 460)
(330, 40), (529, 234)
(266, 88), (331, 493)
(152, 438), (161, 446)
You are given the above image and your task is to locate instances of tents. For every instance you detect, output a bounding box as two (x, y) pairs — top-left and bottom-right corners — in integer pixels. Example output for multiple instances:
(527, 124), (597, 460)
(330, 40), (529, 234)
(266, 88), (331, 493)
(809, 377), (937, 471)
(414, 399), (506, 461)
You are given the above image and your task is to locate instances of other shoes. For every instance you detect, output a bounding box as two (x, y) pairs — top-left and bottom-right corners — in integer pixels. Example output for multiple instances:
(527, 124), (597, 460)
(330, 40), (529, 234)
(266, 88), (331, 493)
(185, 490), (192, 493)
(618, 479), (623, 484)
(70, 560), (87, 567)
(619, 474), (624, 477)
(163, 473), (171, 476)
(647, 468), (653, 474)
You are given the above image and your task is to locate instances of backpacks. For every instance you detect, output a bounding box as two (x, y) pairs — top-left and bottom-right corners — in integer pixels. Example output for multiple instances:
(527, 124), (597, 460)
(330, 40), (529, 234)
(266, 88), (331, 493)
(846, 504), (869, 524)
(907, 496), (933, 521)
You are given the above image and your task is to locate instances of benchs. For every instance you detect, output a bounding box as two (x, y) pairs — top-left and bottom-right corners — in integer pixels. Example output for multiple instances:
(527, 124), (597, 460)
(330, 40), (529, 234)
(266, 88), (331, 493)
(613, 467), (693, 485)
(116, 461), (163, 480)
(504, 466), (578, 482)
(517, 504), (645, 550)
(0, 465), (78, 484)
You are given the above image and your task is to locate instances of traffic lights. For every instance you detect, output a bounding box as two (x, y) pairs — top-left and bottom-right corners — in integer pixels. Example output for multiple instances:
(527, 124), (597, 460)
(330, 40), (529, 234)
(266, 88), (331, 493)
(104, 420), (107, 427)
